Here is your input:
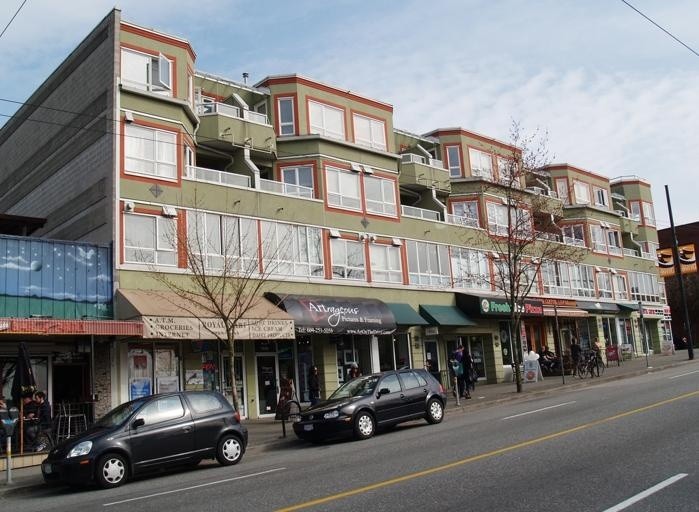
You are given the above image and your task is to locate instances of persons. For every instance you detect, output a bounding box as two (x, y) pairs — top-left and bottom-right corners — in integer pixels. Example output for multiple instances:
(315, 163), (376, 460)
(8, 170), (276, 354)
(539, 337), (583, 377)
(308, 366), (321, 407)
(448, 345), (472, 399)
(0, 391), (53, 452)
(344, 362), (362, 383)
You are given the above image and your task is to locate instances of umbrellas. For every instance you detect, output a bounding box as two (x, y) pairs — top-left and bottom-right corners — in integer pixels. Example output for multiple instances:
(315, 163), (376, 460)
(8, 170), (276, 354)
(11, 342), (38, 455)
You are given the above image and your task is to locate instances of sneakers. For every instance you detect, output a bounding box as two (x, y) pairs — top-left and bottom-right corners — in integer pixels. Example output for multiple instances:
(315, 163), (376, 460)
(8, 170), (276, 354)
(37, 442), (47, 452)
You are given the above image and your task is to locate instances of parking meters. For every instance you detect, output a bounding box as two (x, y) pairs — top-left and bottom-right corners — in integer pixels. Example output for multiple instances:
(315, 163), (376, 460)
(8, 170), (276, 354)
(0, 403), (23, 489)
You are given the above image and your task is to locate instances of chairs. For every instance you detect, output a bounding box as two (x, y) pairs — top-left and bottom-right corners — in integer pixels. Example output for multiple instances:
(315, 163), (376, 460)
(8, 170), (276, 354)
(511, 362), (524, 382)
(0, 406), (59, 452)
(605, 345), (619, 367)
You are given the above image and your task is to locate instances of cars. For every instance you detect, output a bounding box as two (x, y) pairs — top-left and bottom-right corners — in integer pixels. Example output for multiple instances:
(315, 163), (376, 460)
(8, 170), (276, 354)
(292, 368), (448, 440)
(41, 389), (249, 489)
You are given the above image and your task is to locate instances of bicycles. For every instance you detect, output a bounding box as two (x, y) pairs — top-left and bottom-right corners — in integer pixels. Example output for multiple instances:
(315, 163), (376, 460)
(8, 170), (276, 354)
(577, 350), (605, 380)
